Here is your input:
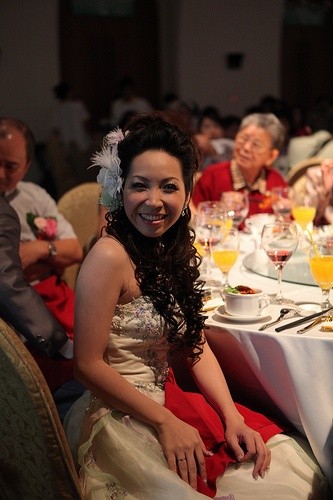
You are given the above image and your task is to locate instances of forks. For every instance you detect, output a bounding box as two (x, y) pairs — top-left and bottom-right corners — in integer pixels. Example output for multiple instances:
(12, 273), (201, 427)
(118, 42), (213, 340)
(258, 308), (299, 331)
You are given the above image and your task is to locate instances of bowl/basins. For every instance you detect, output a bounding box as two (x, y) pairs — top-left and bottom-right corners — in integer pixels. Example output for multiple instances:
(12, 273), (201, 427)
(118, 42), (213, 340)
(220, 287), (269, 316)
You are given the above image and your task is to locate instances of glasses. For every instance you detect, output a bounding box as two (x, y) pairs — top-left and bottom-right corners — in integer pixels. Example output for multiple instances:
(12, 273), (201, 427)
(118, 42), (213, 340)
(235, 131), (272, 153)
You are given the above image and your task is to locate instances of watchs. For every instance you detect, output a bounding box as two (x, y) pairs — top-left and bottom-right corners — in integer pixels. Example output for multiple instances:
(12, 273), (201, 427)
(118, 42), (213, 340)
(48, 241), (56, 258)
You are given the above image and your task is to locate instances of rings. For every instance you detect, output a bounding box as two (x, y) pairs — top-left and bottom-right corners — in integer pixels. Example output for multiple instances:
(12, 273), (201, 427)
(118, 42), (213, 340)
(179, 458), (186, 462)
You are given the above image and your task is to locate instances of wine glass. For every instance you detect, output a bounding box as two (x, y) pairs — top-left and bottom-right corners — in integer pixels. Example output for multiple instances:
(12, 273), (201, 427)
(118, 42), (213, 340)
(309, 240), (333, 316)
(271, 188), (317, 252)
(262, 223), (299, 305)
(195, 190), (249, 290)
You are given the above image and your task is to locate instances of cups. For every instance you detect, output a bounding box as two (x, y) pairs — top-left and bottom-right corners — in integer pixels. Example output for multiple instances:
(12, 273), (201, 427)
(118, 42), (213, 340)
(244, 213), (276, 239)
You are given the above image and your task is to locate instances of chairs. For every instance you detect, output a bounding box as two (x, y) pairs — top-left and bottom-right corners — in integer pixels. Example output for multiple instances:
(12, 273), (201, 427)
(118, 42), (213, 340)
(57, 182), (100, 245)
(0, 317), (85, 500)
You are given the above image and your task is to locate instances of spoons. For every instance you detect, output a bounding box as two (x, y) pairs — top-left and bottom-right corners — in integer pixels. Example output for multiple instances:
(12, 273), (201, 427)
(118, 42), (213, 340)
(297, 315), (333, 333)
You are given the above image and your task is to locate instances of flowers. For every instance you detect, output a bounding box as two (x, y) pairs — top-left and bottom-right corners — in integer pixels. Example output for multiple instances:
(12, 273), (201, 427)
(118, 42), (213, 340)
(26, 207), (57, 241)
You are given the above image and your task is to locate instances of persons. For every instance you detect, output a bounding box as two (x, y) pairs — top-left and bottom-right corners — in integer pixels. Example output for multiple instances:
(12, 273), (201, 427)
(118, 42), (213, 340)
(0, 117), (84, 373)
(261, 95), (312, 138)
(53, 81), (89, 128)
(110, 77), (192, 130)
(193, 109), (235, 170)
(189, 113), (293, 231)
(64, 112), (333, 500)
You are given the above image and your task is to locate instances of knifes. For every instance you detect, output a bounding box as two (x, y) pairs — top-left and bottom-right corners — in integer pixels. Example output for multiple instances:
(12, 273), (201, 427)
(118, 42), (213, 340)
(275, 307), (333, 333)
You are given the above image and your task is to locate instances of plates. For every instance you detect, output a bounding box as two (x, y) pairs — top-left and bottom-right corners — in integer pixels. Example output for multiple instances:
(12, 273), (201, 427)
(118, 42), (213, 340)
(215, 306), (269, 322)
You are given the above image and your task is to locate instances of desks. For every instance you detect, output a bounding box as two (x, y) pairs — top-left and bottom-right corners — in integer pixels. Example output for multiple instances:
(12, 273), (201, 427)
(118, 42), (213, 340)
(187, 215), (333, 483)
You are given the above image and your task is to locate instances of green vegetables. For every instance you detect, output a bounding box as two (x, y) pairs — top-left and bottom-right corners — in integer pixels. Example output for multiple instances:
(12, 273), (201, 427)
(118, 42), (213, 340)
(223, 287), (238, 294)
